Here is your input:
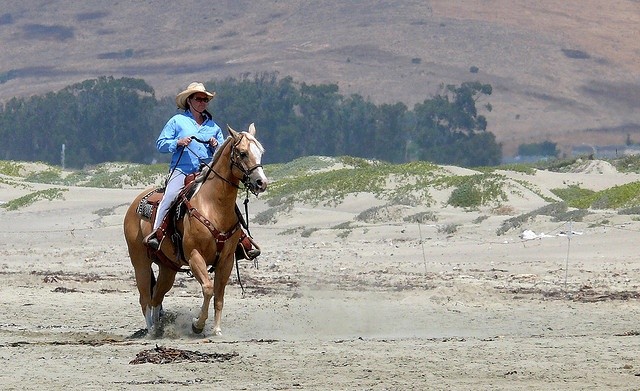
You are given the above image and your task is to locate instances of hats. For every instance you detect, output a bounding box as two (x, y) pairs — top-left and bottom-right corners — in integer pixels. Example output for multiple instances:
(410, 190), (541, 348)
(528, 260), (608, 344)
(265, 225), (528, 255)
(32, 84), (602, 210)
(175, 81), (217, 112)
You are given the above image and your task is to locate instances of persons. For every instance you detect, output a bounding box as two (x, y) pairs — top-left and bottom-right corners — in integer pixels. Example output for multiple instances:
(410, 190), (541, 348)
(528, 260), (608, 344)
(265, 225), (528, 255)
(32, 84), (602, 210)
(146, 82), (261, 260)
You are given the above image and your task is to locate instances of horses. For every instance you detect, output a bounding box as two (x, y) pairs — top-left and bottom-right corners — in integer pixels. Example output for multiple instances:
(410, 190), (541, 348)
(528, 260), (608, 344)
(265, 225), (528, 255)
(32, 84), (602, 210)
(123, 122), (267, 338)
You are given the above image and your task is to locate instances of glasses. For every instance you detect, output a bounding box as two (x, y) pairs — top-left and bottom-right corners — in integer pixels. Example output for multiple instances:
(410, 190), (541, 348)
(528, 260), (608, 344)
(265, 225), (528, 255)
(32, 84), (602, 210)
(192, 97), (209, 103)
